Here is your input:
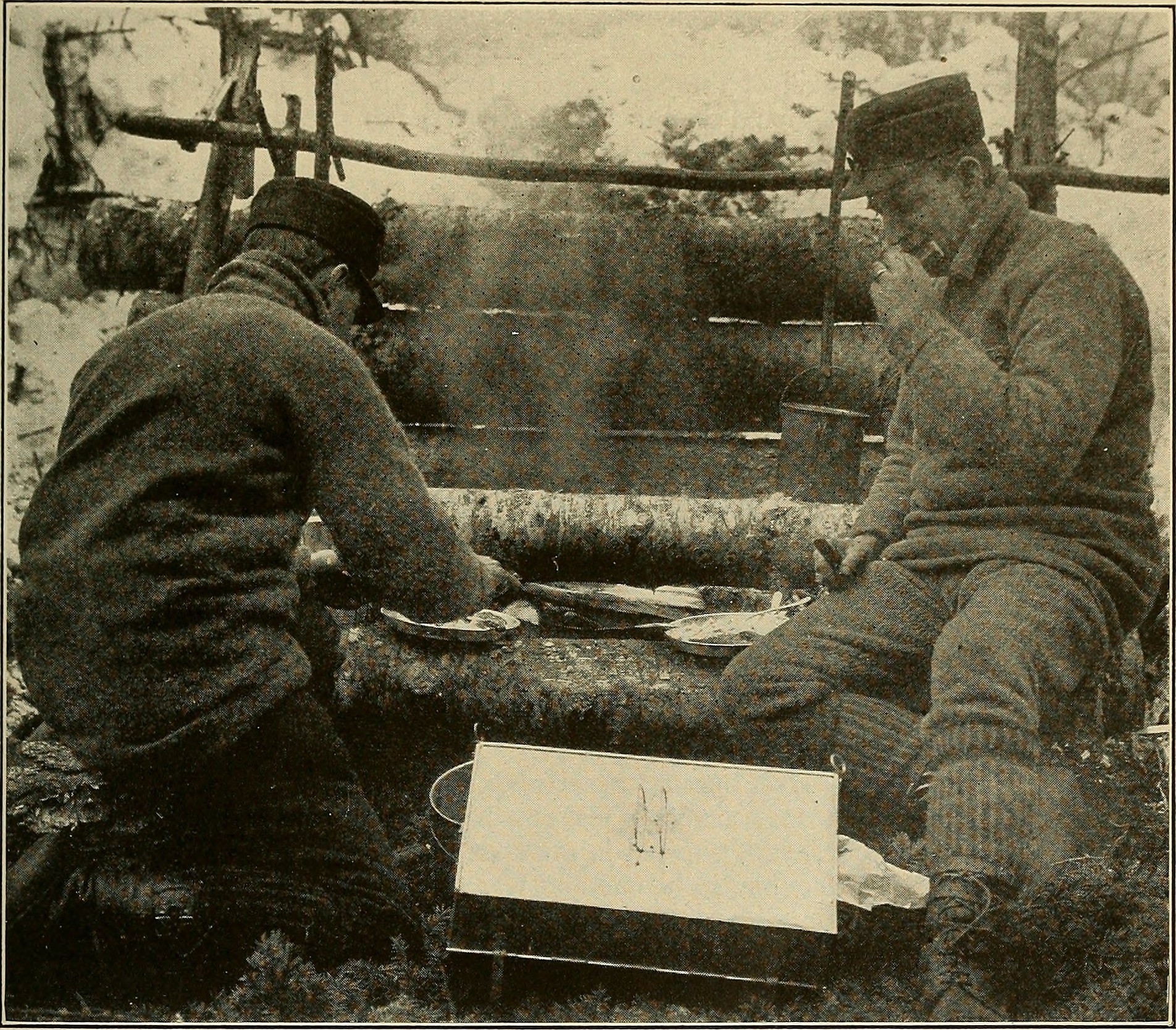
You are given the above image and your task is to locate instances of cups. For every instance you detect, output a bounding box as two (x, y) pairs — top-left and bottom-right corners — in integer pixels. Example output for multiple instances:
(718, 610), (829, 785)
(1137, 723), (1170, 782)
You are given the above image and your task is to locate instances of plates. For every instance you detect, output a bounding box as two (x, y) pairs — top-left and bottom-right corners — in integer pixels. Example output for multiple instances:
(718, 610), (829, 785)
(666, 611), (791, 654)
(381, 602), (522, 643)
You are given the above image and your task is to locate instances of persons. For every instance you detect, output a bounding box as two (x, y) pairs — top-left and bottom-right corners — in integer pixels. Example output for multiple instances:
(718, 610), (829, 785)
(719, 73), (1169, 1020)
(17, 175), (528, 1022)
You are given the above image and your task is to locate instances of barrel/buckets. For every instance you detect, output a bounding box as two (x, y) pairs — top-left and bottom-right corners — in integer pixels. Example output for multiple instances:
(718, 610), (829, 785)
(779, 365), (872, 502)
(426, 758), (475, 912)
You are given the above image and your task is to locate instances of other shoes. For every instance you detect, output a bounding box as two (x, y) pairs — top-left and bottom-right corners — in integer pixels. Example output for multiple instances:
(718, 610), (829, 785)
(4, 833), (83, 952)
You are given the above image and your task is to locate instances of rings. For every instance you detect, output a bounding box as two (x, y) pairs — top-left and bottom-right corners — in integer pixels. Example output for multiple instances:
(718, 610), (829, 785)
(876, 268), (887, 278)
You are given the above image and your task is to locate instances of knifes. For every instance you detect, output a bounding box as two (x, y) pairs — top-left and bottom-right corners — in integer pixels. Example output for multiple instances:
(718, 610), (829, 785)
(812, 536), (852, 588)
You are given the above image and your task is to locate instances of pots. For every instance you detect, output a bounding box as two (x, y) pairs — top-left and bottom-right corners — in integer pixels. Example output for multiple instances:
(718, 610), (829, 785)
(534, 580), (806, 638)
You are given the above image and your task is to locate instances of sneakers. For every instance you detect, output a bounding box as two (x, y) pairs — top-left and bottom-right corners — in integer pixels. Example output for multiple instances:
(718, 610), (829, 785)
(1004, 764), (1085, 912)
(920, 870), (1008, 1022)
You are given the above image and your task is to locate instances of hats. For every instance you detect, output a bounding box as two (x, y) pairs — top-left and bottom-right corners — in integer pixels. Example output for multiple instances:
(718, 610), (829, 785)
(837, 72), (984, 200)
(242, 175), (386, 331)
